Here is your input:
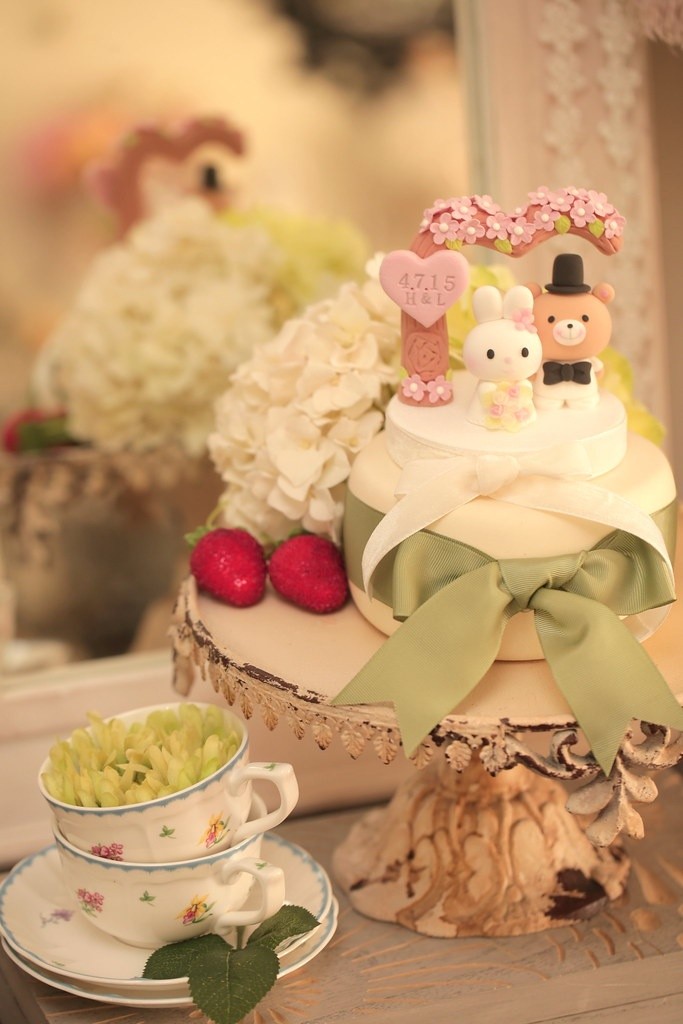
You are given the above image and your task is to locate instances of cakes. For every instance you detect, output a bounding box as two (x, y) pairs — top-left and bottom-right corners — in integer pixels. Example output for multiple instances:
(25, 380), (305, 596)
(341, 185), (679, 663)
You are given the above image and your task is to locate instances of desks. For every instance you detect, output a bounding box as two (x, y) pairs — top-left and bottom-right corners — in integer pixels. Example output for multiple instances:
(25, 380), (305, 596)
(0, 652), (683, 1024)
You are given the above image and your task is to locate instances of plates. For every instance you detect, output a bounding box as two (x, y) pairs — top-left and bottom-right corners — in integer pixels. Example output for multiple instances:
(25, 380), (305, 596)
(1, 831), (333, 991)
(0, 894), (339, 1009)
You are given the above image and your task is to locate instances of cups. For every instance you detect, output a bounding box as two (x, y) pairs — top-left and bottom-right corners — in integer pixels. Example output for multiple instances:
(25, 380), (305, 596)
(36, 701), (300, 863)
(53, 789), (286, 949)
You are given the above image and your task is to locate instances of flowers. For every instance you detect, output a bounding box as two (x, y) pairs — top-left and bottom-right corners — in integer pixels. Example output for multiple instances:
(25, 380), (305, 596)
(29, 197), (371, 492)
(392, 185), (628, 408)
(200, 248), (670, 552)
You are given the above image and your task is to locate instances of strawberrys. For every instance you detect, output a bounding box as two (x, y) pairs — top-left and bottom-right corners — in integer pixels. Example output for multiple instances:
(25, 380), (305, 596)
(189, 527), (267, 606)
(268, 533), (348, 612)
